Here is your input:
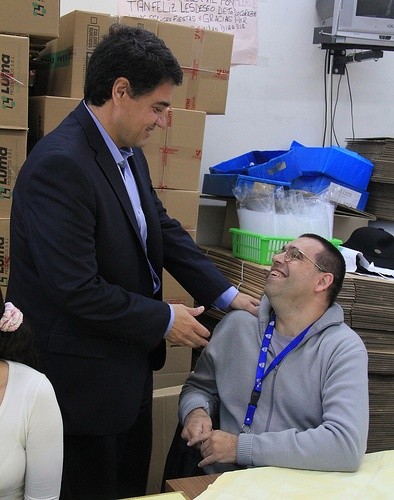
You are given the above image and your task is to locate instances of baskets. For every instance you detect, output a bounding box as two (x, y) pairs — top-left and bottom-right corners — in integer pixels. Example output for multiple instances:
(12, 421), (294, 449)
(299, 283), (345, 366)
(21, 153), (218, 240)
(228, 227), (343, 266)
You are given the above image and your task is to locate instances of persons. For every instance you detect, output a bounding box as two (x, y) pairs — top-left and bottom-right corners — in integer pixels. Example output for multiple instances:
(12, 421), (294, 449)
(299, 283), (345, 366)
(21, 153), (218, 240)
(0, 288), (63, 500)
(5, 22), (260, 500)
(178, 234), (369, 473)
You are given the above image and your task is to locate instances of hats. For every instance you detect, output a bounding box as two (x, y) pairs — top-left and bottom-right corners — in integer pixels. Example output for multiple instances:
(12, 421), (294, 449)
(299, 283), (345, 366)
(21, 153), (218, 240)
(339, 227), (394, 270)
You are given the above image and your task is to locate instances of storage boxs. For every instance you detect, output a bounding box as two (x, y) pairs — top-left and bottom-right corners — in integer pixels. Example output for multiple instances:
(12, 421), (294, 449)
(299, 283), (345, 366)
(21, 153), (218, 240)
(0, 0), (375, 496)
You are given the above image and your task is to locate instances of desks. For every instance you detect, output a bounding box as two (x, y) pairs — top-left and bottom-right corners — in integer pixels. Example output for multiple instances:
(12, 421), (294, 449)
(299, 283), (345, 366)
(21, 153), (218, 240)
(117, 474), (222, 500)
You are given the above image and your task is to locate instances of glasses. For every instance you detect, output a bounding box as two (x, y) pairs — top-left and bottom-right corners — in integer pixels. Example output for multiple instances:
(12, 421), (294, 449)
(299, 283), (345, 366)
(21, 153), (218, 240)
(274, 247), (326, 273)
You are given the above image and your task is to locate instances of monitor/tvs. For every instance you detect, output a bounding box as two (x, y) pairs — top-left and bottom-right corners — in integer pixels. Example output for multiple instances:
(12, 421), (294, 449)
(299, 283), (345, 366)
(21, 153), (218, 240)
(315, 0), (394, 47)
